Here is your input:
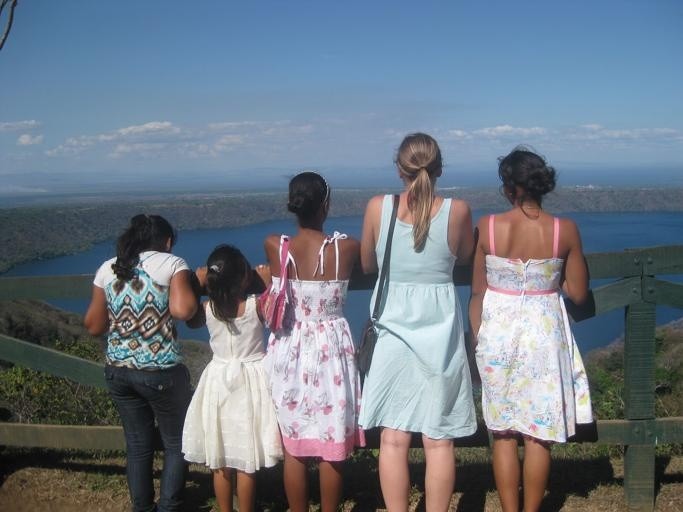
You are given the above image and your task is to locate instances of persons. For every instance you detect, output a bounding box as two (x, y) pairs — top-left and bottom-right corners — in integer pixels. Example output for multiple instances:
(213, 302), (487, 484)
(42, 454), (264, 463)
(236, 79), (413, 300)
(361, 134), (476, 511)
(186, 245), (283, 512)
(263, 172), (366, 512)
(468, 141), (589, 512)
(84, 214), (198, 512)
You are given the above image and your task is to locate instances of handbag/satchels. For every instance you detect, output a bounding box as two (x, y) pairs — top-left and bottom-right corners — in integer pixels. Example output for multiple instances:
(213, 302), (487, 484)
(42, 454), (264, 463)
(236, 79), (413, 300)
(255, 277), (287, 332)
(355, 323), (376, 372)
